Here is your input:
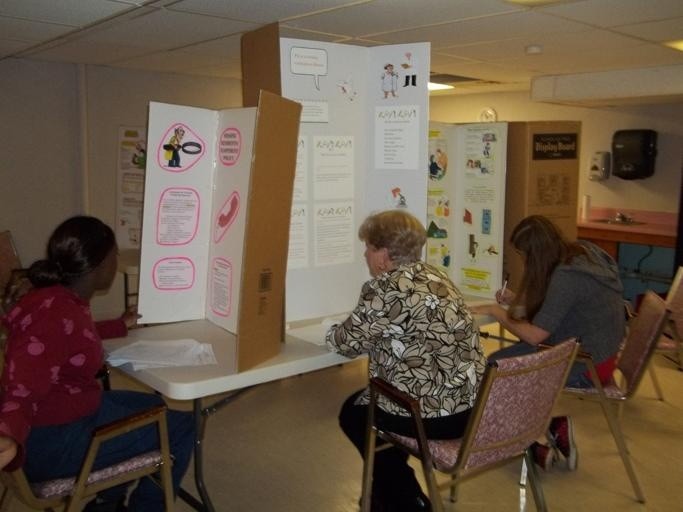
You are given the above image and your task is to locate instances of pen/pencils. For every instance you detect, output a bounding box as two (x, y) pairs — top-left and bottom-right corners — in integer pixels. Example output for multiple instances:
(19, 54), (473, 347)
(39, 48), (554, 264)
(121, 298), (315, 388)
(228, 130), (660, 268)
(498, 273), (511, 303)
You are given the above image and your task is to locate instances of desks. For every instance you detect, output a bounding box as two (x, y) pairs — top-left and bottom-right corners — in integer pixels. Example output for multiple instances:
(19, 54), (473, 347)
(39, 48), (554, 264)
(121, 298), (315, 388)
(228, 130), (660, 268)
(99, 297), (517, 511)
(117, 249), (140, 320)
(579, 212), (682, 298)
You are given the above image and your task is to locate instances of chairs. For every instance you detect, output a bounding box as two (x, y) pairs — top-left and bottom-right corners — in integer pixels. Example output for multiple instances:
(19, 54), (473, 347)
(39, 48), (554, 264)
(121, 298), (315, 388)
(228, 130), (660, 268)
(365, 338), (578, 512)
(2, 407), (173, 511)
(521, 292), (672, 503)
(629, 266), (683, 371)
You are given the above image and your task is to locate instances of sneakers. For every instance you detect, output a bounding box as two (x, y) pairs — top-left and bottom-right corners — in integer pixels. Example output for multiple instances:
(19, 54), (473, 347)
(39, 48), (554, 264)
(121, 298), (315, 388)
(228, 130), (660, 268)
(546, 415), (577, 471)
(531, 442), (554, 472)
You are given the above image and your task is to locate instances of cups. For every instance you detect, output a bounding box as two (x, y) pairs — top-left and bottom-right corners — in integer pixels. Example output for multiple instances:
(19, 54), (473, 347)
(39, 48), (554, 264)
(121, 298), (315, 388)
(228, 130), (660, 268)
(582, 194), (591, 224)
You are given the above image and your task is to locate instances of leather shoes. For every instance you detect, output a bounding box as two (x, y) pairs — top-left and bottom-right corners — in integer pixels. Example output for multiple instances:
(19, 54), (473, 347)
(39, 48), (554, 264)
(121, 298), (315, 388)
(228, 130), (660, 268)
(359, 493), (431, 511)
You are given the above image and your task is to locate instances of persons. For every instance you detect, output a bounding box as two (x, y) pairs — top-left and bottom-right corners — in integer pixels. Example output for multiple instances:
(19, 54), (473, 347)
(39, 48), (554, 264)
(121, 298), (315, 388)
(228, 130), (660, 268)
(401, 51), (420, 89)
(440, 244), (453, 268)
(435, 196), (452, 218)
(163, 126), (191, 167)
(464, 156), (492, 183)
(478, 214), (624, 470)
(0, 206), (198, 512)
(130, 144), (146, 170)
(480, 138), (495, 158)
(326, 209), (491, 512)
(380, 62), (396, 100)
(428, 146), (448, 181)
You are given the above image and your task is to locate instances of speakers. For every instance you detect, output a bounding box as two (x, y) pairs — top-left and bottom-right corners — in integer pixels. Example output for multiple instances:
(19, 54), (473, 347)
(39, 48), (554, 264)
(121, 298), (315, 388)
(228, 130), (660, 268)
(611, 129), (657, 180)
